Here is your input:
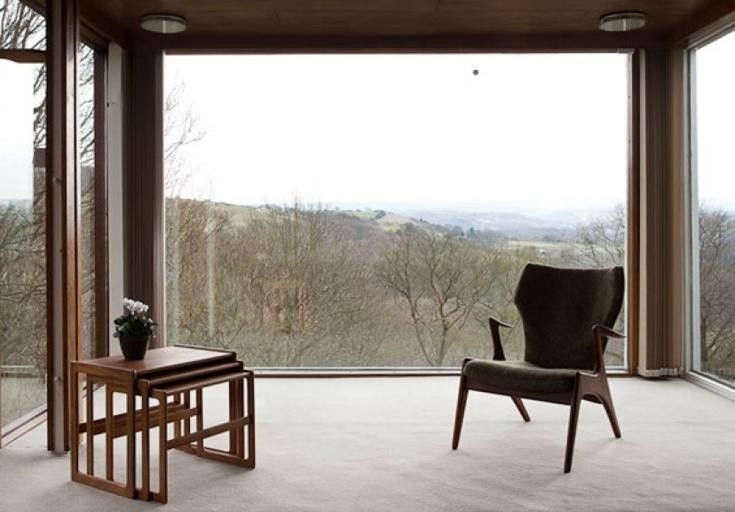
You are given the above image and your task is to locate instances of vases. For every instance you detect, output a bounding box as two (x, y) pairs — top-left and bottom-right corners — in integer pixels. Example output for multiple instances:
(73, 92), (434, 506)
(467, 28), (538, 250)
(118, 334), (149, 360)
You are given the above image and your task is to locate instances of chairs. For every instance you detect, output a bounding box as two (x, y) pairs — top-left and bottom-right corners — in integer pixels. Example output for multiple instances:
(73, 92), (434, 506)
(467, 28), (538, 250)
(453, 264), (627, 473)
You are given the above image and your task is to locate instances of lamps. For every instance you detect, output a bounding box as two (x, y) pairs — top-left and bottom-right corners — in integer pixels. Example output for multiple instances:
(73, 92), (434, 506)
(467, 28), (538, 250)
(599, 12), (646, 32)
(141, 14), (187, 34)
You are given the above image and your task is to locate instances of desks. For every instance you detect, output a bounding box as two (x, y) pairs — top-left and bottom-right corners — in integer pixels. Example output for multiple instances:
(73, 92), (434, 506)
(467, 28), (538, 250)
(71, 344), (255, 504)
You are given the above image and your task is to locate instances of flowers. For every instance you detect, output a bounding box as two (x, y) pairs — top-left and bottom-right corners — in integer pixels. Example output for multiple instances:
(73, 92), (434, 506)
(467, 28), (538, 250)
(113, 297), (158, 342)
